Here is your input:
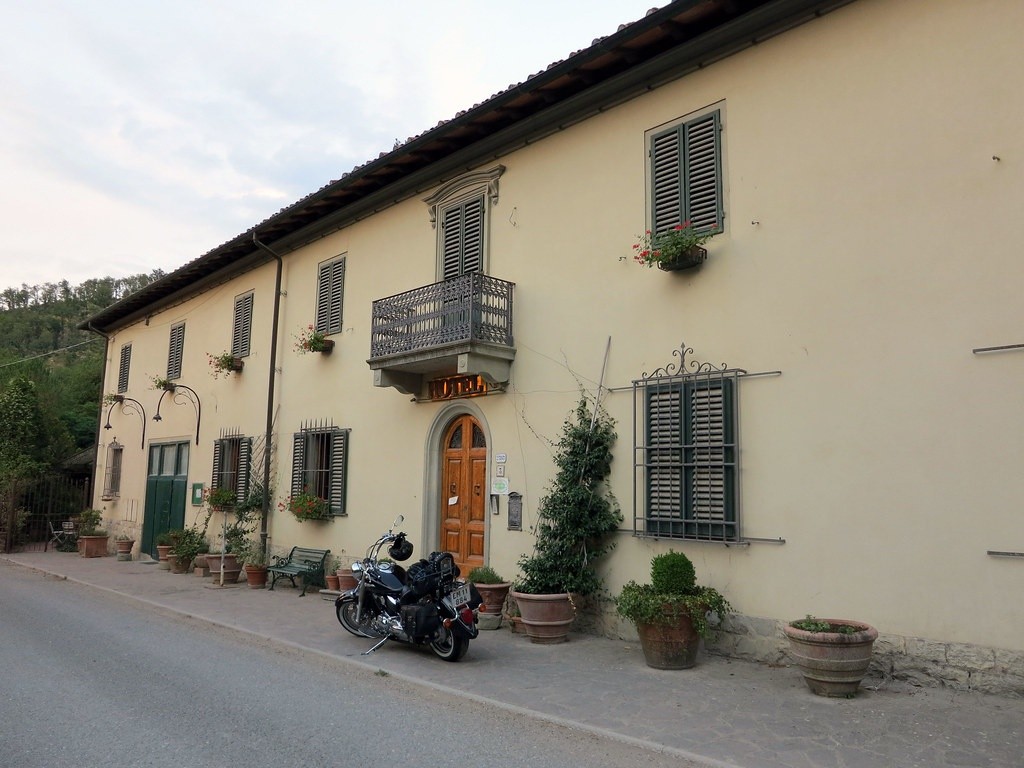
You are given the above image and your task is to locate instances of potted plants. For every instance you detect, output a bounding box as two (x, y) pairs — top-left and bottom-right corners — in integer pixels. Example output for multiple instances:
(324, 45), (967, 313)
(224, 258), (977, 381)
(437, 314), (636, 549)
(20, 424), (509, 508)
(467, 372), (735, 669)
(145, 373), (175, 390)
(79, 508), (110, 557)
(115, 535), (136, 554)
(156, 446), (272, 588)
(327, 548), (394, 590)
(783, 614), (878, 697)
(103, 389), (123, 405)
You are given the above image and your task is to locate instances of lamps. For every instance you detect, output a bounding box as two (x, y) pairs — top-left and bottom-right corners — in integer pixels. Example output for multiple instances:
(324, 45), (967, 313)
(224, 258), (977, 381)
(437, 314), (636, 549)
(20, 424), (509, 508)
(153, 385), (200, 445)
(104, 398), (146, 449)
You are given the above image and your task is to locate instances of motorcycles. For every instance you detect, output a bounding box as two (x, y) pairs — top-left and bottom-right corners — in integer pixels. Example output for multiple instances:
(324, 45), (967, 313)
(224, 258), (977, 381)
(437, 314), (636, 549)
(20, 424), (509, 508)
(334, 513), (487, 663)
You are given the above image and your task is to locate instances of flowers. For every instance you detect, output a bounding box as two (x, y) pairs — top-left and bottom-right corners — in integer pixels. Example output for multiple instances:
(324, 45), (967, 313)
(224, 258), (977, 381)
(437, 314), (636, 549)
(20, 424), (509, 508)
(289, 324), (328, 355)
(202, 482), (236, 512)
(206, 350), (235, 379)
(277, 487), (324, 523)
(633, 220), (717, 269)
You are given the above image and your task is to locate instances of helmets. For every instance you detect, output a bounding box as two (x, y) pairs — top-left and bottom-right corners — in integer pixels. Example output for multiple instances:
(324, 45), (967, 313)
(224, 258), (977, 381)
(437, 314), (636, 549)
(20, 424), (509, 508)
(389, 538), (413, 561)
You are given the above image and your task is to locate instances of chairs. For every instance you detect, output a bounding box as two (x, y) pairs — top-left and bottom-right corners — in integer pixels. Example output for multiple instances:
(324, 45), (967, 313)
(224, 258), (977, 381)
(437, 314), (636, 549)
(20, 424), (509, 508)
(49, 521), (78, 545)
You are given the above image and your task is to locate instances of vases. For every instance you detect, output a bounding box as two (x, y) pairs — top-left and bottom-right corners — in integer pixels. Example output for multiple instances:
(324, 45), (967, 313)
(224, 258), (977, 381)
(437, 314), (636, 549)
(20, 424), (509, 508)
(321, 340), (332, 348)
(662, 245), (707, 269)
(337, 568), (358, 590)
(233, 358), (241, 367)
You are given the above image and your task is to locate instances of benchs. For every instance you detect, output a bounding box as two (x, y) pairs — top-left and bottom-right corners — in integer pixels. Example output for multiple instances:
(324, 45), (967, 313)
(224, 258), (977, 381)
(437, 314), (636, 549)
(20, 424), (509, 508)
(265, 546), (331, 597)
